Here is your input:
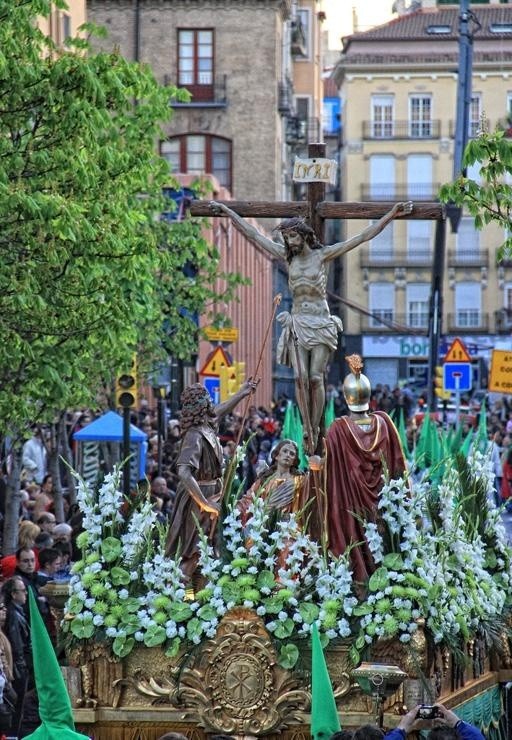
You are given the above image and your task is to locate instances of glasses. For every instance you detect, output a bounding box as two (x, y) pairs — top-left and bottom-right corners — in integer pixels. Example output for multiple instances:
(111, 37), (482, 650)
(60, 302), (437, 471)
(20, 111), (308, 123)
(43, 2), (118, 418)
(15, 588), (26, 592)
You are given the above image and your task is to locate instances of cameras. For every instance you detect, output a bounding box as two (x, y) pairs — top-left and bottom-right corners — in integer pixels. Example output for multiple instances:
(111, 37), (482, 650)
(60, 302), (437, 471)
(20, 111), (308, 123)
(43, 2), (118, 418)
(417, 706), (438, 720)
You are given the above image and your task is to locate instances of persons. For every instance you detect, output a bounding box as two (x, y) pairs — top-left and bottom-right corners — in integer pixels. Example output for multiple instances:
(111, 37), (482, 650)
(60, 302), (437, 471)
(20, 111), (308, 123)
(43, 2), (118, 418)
(208, 198), (415, 457)
(0, 359), (512, 738)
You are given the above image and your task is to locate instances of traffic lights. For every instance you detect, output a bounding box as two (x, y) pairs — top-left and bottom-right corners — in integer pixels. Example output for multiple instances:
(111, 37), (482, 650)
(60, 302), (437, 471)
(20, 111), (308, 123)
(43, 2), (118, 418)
(114, 349), (137, 409)
